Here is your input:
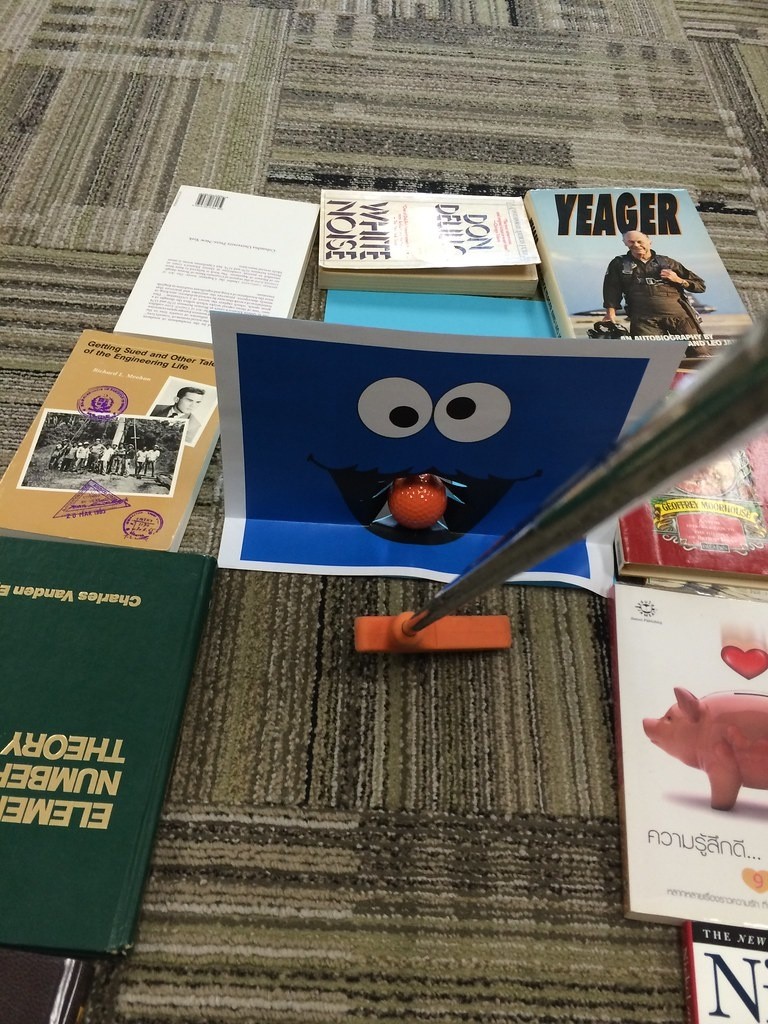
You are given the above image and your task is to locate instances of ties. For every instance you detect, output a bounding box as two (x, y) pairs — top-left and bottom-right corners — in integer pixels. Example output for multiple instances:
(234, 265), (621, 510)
(169, 413), (178, 418)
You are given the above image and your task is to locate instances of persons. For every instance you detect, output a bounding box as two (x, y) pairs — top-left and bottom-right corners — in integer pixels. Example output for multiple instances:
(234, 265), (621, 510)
(598, 228), (712, 361)
(49, 437), (161, 481)
(151, 386), (207, 445)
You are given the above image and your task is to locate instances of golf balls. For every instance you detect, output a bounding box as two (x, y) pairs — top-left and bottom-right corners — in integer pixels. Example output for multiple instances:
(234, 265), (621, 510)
(388, 471), (447, 528)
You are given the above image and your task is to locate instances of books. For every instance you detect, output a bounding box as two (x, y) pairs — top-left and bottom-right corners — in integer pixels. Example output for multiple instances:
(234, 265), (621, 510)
(613, 367), (768, 589)
(112, 183), (321, 349)
(606, 582), (768, 932)
(523, 187), (756, 374)
(0, 532), (229, 960)
(676, 916), (768, 1024)
(0, 948), (104, 1024)
(313, 188), (530, 296)
(0, 324), (223, 558)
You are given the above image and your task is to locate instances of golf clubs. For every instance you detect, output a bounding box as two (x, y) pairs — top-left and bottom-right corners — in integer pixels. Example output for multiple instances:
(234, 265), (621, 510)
(354, 305), (768, 649)
